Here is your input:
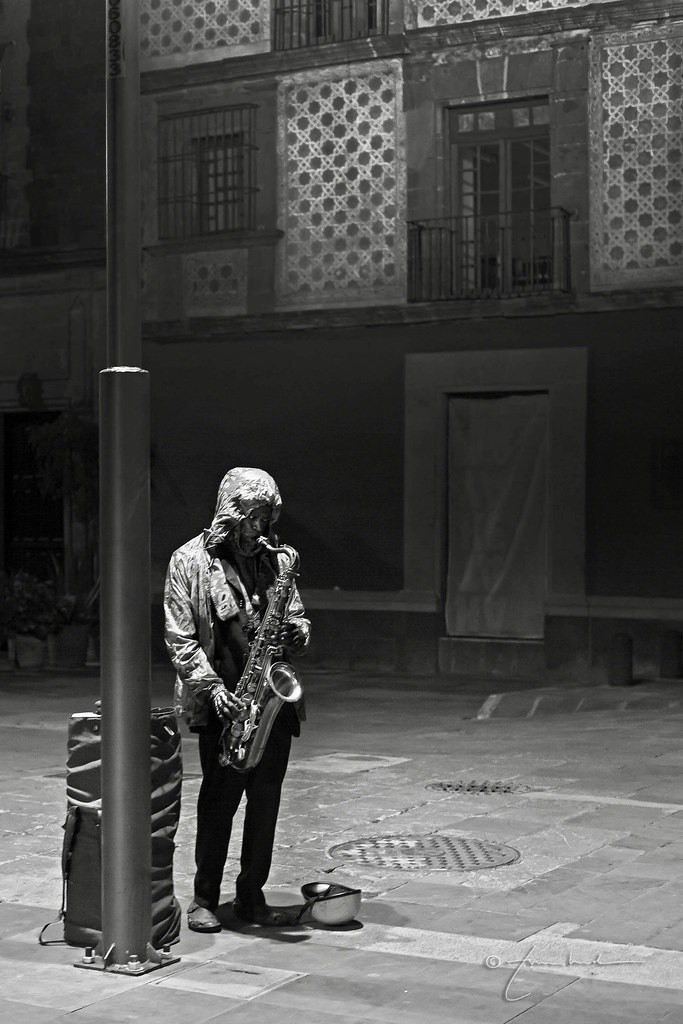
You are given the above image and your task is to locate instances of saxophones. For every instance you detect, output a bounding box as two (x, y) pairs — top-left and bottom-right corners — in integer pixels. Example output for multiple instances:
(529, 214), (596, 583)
(217, 535), (305, 774)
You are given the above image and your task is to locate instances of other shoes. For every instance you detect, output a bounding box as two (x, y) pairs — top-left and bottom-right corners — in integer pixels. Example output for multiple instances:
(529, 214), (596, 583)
(230, 898), (287, 926)
(187, 900), (222, 930)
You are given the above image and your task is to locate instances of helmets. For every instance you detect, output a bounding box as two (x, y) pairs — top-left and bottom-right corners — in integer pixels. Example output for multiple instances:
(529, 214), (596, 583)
(301, 881), (362, 926)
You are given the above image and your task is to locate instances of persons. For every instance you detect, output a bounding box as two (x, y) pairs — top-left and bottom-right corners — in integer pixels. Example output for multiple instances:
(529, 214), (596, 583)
(164, 466), (310, 930)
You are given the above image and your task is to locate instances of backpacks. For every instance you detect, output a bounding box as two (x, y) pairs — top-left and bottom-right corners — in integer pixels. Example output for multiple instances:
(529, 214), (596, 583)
(60, 706), (181, 951)
